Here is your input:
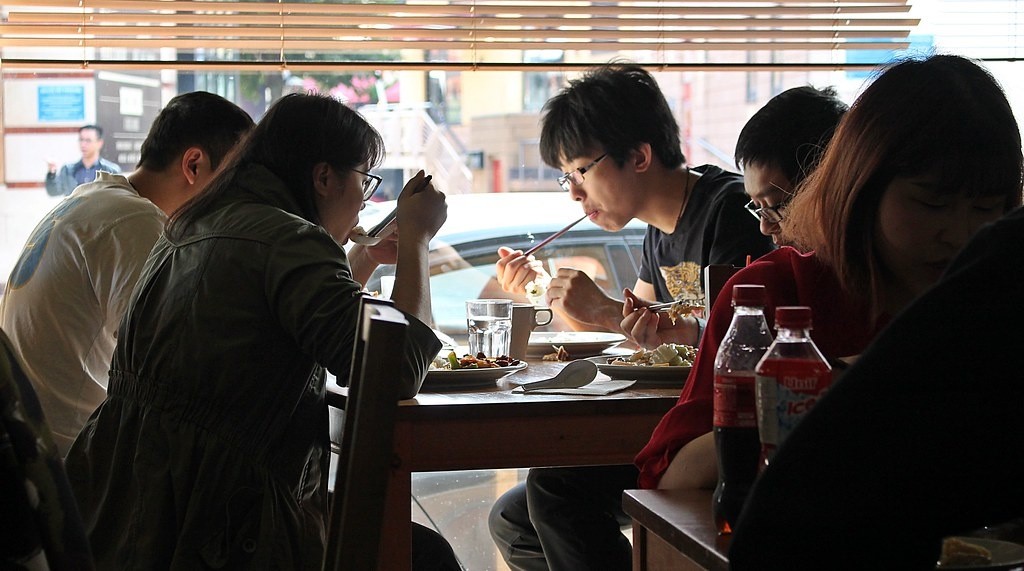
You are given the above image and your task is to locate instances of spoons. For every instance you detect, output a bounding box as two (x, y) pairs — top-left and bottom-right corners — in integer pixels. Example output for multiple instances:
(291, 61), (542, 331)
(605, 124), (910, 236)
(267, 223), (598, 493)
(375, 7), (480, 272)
(522, 360), (597, 391)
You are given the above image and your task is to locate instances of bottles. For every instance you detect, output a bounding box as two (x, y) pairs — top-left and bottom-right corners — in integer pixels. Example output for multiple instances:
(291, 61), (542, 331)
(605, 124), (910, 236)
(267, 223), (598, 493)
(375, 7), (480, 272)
(755, 305), (834, 482)
(710, 284), (774, 533)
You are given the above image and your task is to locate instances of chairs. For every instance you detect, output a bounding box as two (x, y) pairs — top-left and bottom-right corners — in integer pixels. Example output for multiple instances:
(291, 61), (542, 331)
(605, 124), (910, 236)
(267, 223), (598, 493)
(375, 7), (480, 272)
(0, 291), (415, 571)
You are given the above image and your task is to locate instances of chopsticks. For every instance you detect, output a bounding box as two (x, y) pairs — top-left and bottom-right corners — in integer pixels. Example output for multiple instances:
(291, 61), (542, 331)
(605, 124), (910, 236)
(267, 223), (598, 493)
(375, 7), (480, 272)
(522, 215), (587, 256)
(365, 175), (432, 237)
(633, 299), (683, 313)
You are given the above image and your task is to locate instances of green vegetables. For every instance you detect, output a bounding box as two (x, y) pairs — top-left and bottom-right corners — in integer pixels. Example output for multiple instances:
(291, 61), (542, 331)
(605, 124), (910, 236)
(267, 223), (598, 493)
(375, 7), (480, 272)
(670, 347), (694, 366)
(448, 351), (479, 369)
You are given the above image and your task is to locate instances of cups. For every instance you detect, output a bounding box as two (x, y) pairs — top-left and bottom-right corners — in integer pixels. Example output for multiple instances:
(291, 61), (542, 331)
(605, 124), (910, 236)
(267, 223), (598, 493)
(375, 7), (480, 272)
(466, 299), (513, 357)
(510, 303), (553, 360)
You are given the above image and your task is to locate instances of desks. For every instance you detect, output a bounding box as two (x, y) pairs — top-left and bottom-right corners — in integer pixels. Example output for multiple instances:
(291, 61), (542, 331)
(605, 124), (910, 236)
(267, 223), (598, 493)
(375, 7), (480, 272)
(325, 333), (684, 571)
(621, 487), (735, 571)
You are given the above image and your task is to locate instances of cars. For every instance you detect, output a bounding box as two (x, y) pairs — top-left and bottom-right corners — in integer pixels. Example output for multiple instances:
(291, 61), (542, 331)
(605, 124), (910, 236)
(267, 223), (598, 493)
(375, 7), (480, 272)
(327, 191), (650, 571)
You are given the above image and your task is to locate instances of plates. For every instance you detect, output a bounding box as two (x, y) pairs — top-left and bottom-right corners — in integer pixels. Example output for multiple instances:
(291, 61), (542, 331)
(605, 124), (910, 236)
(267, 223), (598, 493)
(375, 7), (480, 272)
(425, 360), (528, 387)
(525, 331), (628, 357)
(937, 536), (1024, 571)
(597, 363), (692, 382)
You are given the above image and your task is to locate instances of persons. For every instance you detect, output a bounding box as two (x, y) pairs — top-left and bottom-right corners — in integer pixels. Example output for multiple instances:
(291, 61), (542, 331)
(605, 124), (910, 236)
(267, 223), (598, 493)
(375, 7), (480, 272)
(0, 91), (258, 465)
(736, 86), (846, 253)
(46, 125), (121, 198)
(725, 205), (1024, 571)
(634, 54), (1024, 492)
(60, 92), (461, 570)
(488, 67), (777, 571)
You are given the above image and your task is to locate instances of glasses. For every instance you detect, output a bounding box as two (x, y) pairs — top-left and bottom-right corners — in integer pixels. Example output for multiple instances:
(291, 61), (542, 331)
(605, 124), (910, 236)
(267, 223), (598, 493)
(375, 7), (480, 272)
(556, 156), (613, 192)
(743, 199), (790, 223)
(331, 164), (383, 201)
